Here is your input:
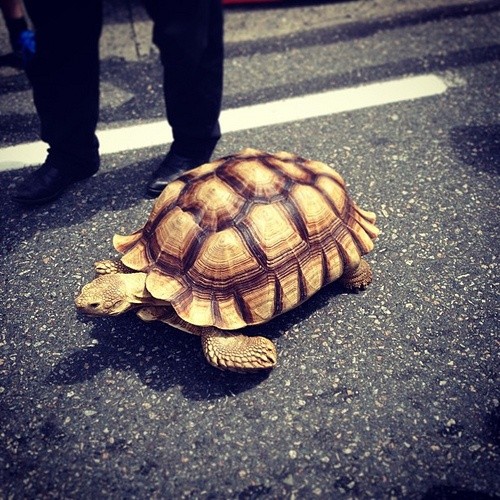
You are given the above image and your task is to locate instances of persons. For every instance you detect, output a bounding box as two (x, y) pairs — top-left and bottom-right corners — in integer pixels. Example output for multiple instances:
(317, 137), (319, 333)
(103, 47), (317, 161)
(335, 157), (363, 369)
(0, 0), (224, 204)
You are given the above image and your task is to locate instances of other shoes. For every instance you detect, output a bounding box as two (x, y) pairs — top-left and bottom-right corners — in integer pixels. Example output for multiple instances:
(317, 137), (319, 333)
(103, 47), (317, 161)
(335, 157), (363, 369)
(10, 25), (37, 69)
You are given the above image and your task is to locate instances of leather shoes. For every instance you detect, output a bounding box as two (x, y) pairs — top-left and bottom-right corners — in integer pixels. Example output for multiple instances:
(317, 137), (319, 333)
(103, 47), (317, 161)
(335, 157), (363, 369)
(11, 153), (100, 204)
(147, 149), (209, 195)
